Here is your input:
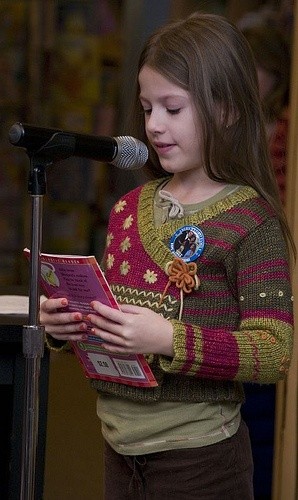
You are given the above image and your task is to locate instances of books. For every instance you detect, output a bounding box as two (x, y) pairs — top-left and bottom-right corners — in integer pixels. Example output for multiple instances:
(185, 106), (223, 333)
(22, 247), (160, 390)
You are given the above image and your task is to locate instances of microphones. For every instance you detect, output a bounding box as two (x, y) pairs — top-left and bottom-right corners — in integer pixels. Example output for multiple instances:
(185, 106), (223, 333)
(8, 122), (150, 171)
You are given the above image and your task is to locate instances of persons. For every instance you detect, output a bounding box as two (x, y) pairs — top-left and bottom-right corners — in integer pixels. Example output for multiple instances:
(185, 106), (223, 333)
(230, 15), (290, 208)
(38, 11), (297, 500)
